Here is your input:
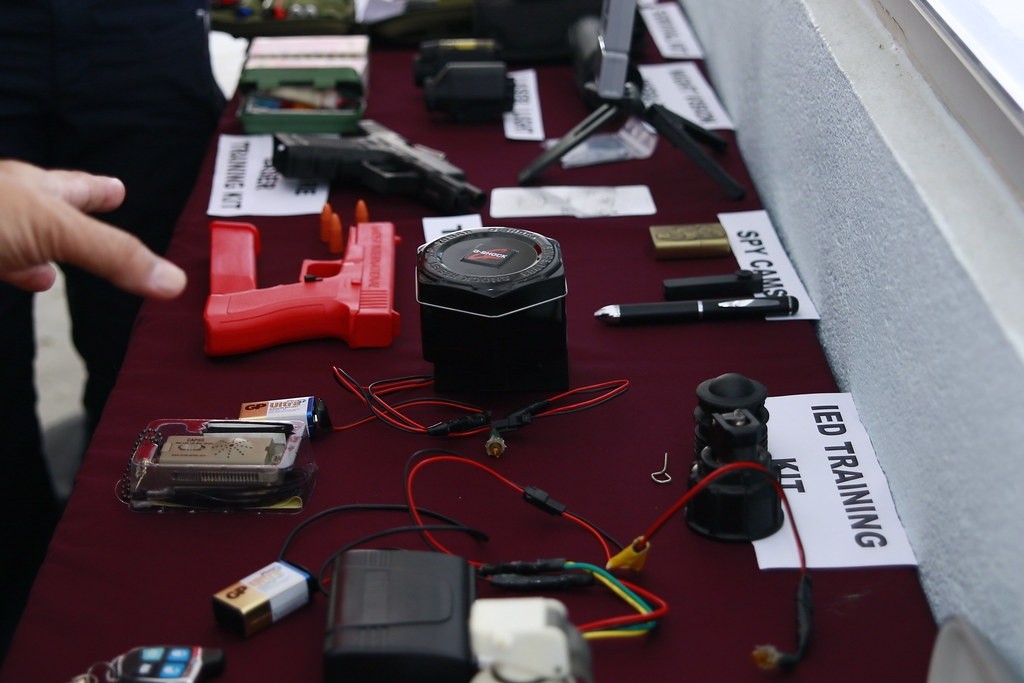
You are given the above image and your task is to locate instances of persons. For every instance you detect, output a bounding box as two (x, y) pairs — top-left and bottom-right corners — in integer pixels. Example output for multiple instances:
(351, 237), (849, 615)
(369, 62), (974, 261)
(0, 152), (188, 300)
(0, 2), (219, 663)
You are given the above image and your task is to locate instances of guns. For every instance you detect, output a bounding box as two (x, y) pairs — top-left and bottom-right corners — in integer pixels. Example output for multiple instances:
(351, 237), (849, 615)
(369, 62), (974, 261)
(204, 216), (404, 358)
(274, 118), (486, 213)
